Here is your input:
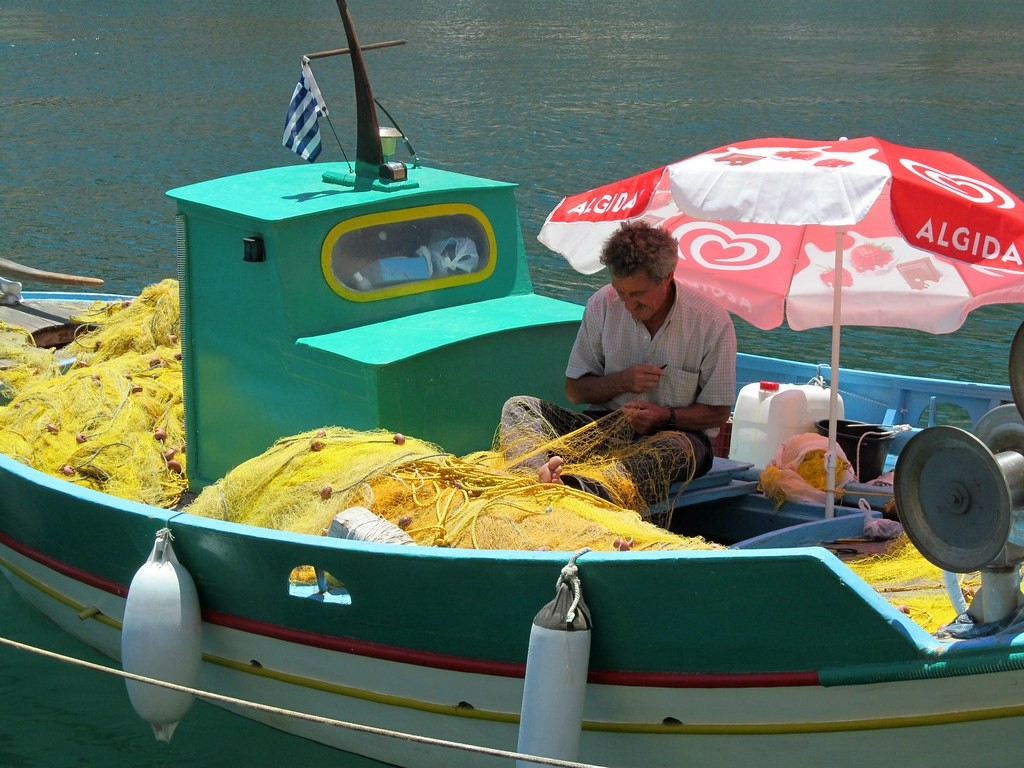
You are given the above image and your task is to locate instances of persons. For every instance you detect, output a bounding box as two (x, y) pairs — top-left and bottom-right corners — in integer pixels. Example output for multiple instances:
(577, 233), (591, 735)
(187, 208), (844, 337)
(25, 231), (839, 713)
(497, 221), (737, 488)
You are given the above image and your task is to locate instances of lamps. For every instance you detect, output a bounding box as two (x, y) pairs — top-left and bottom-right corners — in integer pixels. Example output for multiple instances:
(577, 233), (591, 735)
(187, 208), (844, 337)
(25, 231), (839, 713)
(379, 126), (403, 163)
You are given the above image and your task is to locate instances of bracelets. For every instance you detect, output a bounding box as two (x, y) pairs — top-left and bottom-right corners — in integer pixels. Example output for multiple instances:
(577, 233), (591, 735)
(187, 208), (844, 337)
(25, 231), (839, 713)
(665, 405), (677, 430)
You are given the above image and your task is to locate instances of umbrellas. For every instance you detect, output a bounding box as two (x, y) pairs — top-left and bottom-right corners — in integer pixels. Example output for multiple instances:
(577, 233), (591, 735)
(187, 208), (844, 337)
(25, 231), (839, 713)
(537, 137), (1024, 520)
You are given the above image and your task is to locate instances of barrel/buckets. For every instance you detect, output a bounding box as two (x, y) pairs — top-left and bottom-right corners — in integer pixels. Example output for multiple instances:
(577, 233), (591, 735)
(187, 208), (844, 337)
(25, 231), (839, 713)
(729, 381), (844, 470)
(815, 419), (912, 484)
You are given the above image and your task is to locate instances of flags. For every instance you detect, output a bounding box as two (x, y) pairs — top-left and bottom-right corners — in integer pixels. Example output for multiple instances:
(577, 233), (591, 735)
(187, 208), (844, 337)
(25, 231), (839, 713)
(280, 56), (328, 163)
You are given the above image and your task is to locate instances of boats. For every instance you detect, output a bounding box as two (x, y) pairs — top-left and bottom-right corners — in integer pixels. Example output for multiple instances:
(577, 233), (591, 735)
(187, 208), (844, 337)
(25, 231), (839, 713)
(0, 1), (1023, 768)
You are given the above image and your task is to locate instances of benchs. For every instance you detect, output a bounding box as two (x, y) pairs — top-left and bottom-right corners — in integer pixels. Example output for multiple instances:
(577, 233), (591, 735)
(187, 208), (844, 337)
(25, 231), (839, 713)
(644, 456), (762, 516)
